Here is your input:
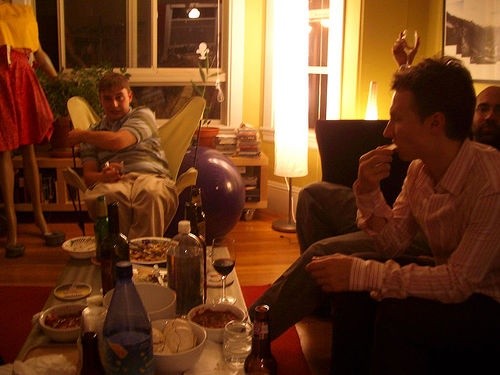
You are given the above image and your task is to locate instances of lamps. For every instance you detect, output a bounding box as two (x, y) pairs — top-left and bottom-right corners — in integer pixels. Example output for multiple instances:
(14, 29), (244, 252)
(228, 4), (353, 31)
(267, 0), (310, 231)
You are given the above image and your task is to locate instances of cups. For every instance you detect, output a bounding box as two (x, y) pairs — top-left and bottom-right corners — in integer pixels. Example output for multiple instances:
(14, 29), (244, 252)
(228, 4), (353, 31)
(105, 161), (124, 179)
(82, 305), (107, 344)
(223, 320), (253, 369)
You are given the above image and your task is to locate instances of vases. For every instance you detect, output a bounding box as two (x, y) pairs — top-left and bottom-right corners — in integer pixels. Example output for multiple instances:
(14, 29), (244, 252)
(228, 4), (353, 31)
(195, 63), (219, 151)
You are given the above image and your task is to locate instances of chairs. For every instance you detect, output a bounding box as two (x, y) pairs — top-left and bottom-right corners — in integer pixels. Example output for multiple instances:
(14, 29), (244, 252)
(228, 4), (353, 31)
(67, 95), (207, 234)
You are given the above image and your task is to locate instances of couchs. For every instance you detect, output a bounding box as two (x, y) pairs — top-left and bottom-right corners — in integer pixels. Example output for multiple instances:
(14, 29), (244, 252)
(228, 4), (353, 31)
(295, 117), (409, 255)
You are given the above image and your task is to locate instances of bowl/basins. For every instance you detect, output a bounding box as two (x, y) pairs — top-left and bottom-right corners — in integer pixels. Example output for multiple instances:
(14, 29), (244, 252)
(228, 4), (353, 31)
(102, 284), (177, 320)
(39, 303), (89, 341)
(150, 317), (207, 373)
(62, 236), (96, 258)
(187, 303), (248, 343)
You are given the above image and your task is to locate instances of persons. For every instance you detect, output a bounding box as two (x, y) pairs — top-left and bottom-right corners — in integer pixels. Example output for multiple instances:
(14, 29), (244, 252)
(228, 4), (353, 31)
(65, 73), (179, 240)
(0, 0), (63, 260)
(245, 29), (500, 375)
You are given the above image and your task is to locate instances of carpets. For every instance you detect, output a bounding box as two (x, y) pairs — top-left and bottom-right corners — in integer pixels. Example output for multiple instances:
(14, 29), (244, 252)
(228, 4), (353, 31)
(0, 283), (308, 375)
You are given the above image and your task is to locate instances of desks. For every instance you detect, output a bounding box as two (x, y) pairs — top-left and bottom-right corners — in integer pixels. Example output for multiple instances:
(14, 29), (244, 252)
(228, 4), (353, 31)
(0, 154), (95, 214)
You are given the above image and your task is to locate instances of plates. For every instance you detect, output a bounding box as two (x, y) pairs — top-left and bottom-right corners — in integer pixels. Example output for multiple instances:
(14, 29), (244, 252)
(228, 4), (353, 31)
(129, 237), (172, 264)
(131, 264), (168, 284)
(53, 283), (92, 302)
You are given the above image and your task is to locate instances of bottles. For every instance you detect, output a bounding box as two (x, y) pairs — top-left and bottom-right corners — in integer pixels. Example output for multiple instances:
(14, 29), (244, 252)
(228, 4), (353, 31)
(191, 188), (203, 212)
(101, 202), (129, 296)
(105, 260), (153, 375)
(244, 304), (278, 375)
(78, 332), (105, 375)
(94, 195), (109, 265)
(165, 220), (205, 311)
(185, 201), (207, 303)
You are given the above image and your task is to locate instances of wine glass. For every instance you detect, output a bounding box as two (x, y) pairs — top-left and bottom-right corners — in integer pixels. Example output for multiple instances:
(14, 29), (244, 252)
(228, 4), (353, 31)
(212, 238), (237, 304)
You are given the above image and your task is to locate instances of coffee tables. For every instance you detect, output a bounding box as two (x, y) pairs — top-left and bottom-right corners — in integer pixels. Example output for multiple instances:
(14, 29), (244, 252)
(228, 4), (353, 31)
(17, 242), (257, 375)
